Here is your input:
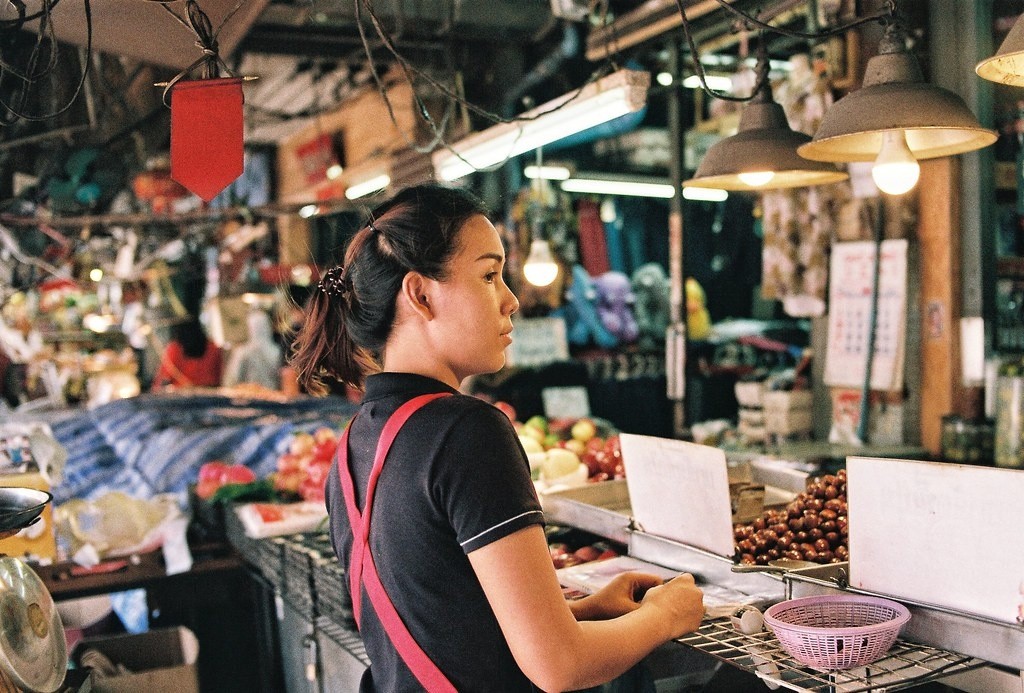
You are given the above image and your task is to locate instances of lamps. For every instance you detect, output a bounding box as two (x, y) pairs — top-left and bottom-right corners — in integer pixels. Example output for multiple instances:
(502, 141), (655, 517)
(430, 69), (651, 183)
(681, 27), (850, 192)
(975, 12), (1024, 87)
(797, 0), (999, 194)
(562, 171), (730, 203)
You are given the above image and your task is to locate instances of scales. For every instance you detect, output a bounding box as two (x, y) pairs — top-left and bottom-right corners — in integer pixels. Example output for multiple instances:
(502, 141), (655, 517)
(0, 486), (95, 693)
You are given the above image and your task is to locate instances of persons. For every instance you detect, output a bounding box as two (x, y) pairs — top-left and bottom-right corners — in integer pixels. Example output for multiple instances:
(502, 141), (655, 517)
(150, 315), (224, 390)
(288, 181), (706, 693)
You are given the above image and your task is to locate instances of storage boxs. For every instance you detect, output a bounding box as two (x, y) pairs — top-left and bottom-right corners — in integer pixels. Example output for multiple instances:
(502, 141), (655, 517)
(70, 625), (201, 693)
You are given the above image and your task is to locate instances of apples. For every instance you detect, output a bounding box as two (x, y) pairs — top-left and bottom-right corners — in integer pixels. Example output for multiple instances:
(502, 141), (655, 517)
(494, 402), (629, 486)
(195, 427), (343, 501)
(548, 542), (621, 571)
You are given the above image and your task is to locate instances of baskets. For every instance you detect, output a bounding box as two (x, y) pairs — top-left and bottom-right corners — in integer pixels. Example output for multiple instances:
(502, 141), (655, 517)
(763, 592), (914, 671)
